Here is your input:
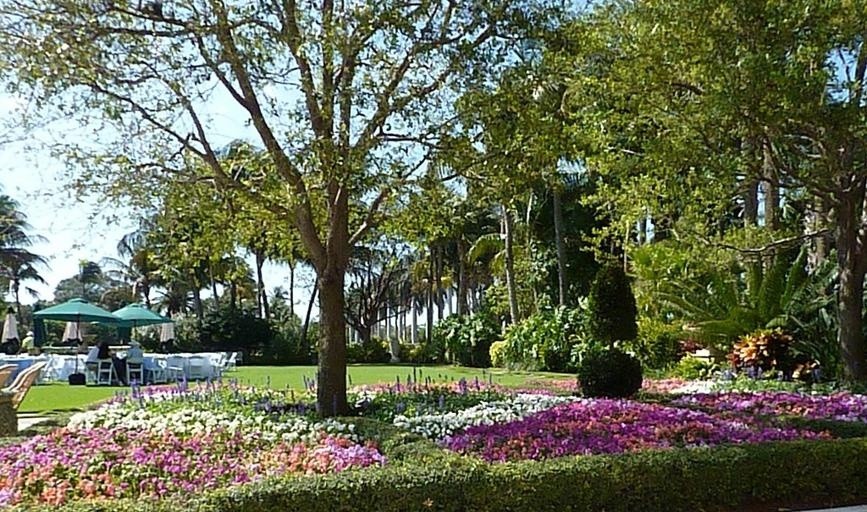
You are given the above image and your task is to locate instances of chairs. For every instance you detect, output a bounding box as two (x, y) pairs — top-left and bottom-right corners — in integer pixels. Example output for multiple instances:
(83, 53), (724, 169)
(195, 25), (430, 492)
(0, 337), (237, 410)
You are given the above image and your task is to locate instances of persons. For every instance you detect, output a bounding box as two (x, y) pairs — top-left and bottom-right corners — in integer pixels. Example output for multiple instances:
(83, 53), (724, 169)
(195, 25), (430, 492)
(84, 340), (145, 387)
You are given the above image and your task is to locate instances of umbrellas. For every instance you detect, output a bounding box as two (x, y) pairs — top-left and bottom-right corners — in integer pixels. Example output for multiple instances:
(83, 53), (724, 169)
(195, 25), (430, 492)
(1, 297), (175, 373)
